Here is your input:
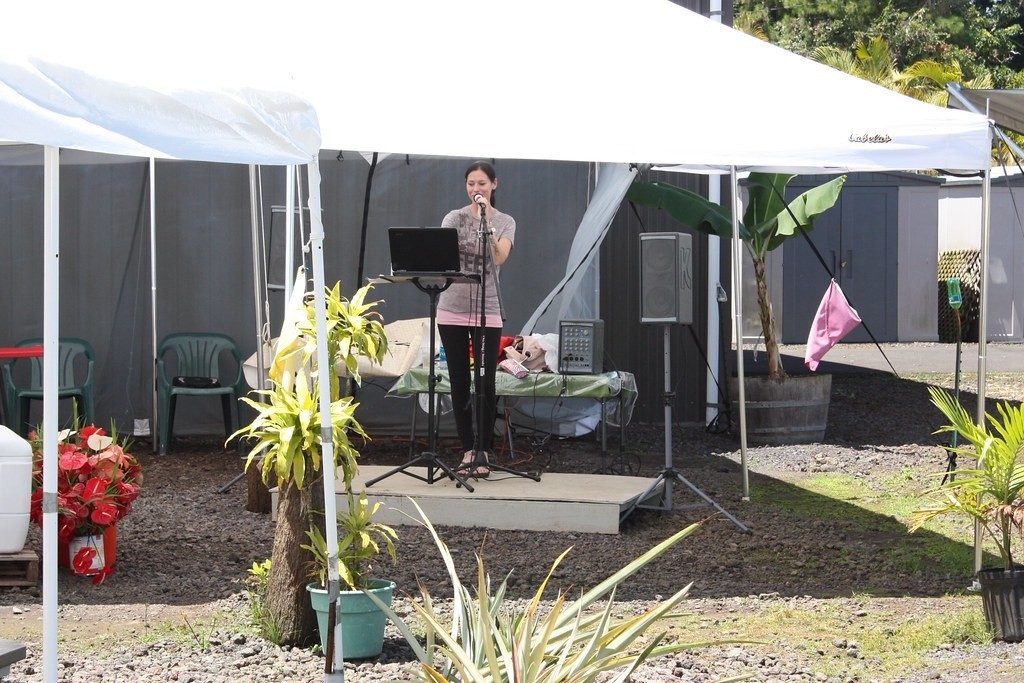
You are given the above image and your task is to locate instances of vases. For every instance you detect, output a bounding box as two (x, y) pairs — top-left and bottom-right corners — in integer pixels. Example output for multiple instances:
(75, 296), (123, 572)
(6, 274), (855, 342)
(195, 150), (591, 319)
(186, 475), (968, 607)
(58, 521), (117, 576)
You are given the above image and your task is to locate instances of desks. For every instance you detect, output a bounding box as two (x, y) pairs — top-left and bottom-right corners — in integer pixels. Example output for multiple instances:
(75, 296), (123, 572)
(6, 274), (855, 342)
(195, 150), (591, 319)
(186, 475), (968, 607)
(385, 362), (638, 473)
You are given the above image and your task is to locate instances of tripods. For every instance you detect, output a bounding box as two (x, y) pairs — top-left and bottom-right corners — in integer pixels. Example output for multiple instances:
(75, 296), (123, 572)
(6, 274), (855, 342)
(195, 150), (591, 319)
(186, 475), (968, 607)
(365, 207), (540, 492)
(620, 325), (754, 535)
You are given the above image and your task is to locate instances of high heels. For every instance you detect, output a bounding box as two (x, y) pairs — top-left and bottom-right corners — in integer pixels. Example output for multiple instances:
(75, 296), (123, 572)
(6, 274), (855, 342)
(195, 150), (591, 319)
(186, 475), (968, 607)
(457, 452), (477, 475)
(473, 453), (490, 477)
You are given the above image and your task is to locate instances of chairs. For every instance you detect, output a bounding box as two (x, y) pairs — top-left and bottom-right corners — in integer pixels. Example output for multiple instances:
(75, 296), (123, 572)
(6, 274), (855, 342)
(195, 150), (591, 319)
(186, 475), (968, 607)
(2, 337), (97, 436)
(155, 333), (246, 461)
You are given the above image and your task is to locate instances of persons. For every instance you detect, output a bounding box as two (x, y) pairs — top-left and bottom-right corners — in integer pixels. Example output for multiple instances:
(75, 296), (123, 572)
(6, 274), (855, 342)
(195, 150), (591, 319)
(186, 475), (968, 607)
(439, 161), (516, 478)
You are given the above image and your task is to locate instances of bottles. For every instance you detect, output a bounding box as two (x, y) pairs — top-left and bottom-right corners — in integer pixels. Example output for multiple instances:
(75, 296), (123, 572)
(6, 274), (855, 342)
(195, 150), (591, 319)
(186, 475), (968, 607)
(439, 339), (449, 370)
(947, 278), (962, 310)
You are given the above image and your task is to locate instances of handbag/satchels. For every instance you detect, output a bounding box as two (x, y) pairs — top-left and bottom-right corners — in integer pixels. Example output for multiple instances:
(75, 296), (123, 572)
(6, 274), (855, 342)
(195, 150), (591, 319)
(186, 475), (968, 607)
(504, 333), (547, 371)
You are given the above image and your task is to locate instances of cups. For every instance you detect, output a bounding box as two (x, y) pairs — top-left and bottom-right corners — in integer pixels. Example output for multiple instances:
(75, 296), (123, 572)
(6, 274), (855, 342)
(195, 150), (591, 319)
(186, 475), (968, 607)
(421, 347), (430, 368)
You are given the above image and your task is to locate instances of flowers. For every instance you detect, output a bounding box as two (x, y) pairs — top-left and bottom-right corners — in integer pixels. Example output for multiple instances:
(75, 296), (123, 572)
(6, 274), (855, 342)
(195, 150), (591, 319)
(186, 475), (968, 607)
(21, 411), (146, 586)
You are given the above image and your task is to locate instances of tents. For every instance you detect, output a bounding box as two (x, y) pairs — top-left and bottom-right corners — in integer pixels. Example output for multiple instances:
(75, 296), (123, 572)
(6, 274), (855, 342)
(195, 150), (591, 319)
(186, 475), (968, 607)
(0, 3), (994, 683)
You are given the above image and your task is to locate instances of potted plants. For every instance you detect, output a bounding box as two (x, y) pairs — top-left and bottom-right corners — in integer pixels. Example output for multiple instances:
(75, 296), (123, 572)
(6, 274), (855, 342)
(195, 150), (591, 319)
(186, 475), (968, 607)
(222, 278), (400, 660)
(907, 385), (1024, 644)
(620, 170), (848, 444)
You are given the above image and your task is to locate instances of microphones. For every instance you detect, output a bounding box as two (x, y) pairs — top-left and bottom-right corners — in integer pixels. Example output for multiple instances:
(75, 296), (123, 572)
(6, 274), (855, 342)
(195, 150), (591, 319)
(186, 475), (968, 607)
(473, 193), (485, 209)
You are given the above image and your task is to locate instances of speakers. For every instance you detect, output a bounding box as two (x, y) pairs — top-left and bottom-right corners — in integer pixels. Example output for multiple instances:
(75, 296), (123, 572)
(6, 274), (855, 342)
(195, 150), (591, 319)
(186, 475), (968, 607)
(640, 232), (692, 325)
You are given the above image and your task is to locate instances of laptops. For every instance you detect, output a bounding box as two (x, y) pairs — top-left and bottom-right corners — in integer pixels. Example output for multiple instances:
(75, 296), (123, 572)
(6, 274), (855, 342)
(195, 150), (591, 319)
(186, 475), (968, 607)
(388, 227), (469, 279)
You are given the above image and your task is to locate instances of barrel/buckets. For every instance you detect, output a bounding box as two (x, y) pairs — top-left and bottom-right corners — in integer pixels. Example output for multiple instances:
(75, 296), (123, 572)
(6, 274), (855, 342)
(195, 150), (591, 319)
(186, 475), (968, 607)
(0, 423), (32, 553)
